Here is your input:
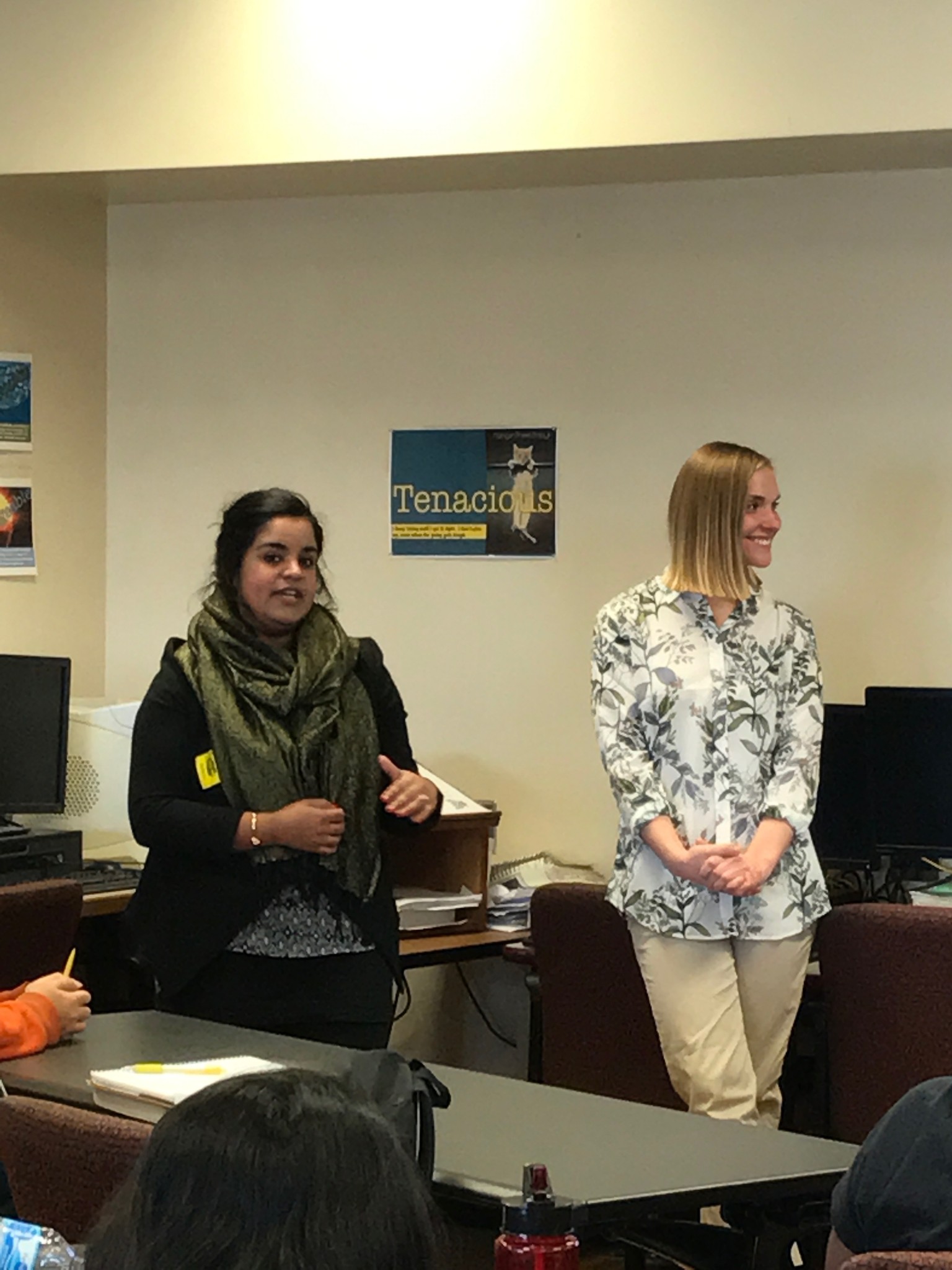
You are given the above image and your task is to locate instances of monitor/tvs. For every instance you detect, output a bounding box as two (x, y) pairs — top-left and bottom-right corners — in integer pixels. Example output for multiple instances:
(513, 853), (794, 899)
(809, 684), (952, 869)
(0, 655), (71, 814)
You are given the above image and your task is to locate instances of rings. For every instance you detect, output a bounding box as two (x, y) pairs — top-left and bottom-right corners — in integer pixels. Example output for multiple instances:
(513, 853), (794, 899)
(419, 794), (430, 804)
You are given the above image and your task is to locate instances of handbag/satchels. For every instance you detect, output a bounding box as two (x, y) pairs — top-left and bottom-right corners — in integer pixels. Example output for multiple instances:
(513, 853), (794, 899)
(339, 1049), (451, 1184)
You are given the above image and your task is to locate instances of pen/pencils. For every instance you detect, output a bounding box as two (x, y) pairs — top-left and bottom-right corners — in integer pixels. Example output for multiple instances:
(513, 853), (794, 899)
(60, 947), (77, 978)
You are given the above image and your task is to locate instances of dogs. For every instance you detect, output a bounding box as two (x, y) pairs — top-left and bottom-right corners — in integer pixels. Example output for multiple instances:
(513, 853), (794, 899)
(508, 444), (539, 544)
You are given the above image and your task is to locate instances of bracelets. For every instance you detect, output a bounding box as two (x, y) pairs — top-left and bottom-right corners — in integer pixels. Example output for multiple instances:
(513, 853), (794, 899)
(249, 809), (262, 848)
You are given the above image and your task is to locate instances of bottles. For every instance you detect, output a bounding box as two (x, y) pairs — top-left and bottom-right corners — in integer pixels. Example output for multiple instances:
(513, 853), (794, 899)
(0, 1215), (85, 1270)
(494, 1164), (581, 1270)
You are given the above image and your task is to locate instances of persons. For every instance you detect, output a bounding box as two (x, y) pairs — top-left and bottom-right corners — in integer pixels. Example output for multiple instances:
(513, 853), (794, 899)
(113, 485), (444, 1051)
(84, 1065), (461, 1269)
(2, 970), (92, 1059)
(588, 440), (836, 1224)
(825, 1068), (951, 1266)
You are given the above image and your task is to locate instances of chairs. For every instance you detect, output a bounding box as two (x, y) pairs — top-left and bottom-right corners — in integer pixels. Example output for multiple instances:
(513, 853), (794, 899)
(0, 1093), (153, 1250)
(808, 901), (952, 1144)
(529, 882), (688, 1115)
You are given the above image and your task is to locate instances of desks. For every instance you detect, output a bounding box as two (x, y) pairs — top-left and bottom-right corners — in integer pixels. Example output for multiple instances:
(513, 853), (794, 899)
(1, 1008), (867, 1270)
